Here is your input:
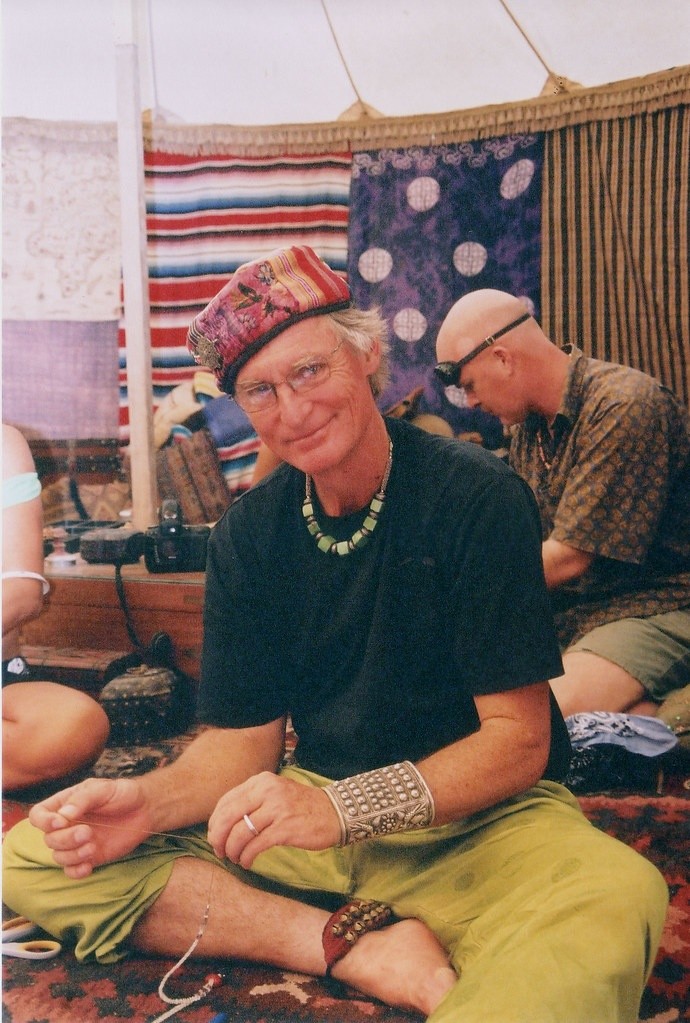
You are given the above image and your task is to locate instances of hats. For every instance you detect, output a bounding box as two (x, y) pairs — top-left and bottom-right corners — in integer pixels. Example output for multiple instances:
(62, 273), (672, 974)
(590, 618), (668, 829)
(186, 245), (351, 398)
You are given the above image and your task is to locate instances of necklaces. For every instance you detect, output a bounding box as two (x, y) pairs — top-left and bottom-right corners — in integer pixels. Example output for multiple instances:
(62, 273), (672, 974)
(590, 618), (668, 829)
(302, 436), (393, 558)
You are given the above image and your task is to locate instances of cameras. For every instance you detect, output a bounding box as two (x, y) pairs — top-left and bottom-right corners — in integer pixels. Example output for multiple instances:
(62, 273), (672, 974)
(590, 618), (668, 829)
(142, 499), (212, 576)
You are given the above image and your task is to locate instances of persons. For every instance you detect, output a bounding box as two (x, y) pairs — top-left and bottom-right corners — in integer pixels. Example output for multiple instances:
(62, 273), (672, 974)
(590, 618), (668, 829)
(2, 245), (669, 1023)
(433, 288), (690, 720)
(0, 423), (110, 791)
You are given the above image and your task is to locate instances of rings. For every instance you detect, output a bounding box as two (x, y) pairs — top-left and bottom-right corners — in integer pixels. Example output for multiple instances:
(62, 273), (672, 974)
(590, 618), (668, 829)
(243, 815), (258, 835)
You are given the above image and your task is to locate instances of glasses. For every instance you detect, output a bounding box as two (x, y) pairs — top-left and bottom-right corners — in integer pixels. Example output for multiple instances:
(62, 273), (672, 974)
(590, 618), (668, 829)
(229, 339), (347, 412)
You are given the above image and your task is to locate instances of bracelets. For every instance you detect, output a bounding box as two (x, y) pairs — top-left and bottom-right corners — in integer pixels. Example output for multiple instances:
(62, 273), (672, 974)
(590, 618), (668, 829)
(321, 761), (435, 849)
